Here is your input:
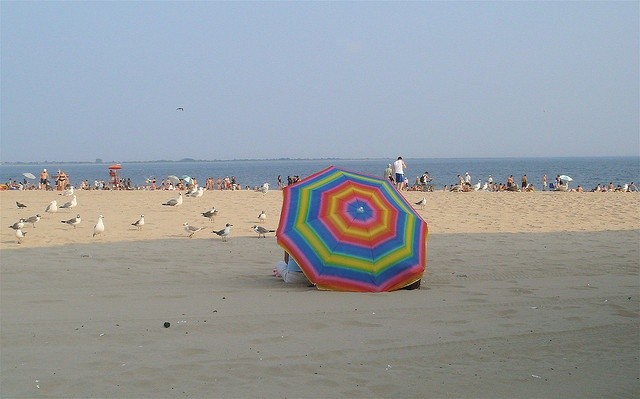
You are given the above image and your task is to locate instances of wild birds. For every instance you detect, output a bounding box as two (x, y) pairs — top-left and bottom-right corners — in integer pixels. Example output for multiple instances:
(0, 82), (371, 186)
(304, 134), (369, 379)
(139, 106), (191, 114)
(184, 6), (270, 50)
(132, 214), (145, 231)
(415, 198), (427, 209)
(203, 210), (218, 222)
(61, 213), (81, 229)
(16, 201), (28, 209)
(162, 191), (183, 207)
(92, 215), (105, 237)
(258, 211), (267, 222)
(59, 195), (78, 210)
(176, 107), (185, 112)
(186, 184), (198, 197)
(212, 223), (233, 242)
(10, 218), (27, 229)
(16, 226), (27, 244)
(192, 187), (204, 198)
(201, 206), (215, 218)
(25, 214), (41, 228)
(58, 185), (75, 197)
(45, 200), (58, 213)
(183, 222), (206, 238)
(259, 183), (269, 196)
(251, 225), (276, 239)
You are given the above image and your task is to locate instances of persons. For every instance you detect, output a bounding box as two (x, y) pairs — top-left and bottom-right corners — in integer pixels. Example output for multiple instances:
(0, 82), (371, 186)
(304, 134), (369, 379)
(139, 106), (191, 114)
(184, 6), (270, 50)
(277, 174), (302, 190)
(465, 172), (472, 185)
(170, 178), (198, 190)
(39, 169), (110, 190)
(571, 180), (640, 192)
(542, 175), (547, 191)
(206, 175), (260, 190)
(488, 174), (494, 191)
(276, 251), (312, 284)
(507, 174), (513, 188)
(384, 163), (396, 187)
(444, 181), (562, 191)
(404, 168), (435, 191)
(393, 157), (406, 190)
(553, 178), (558, 189)
(557, 175), (561, 188)
(5, 177), (27, 190)
(110, 170), (131, 190)
(27, 181), (40, 190)
(143, 177), (170, 191)
(521, 174), (528, 191)
(457, 174), (465, 187)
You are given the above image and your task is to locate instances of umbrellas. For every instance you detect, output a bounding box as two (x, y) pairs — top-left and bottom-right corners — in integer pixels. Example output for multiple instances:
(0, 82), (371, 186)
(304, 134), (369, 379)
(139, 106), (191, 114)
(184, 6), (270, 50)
(181, 174), (195, 186)
(108, 164), (123, 174)
(558, 174), (574, 183)
(22, 172), (36, 181)
(275, 165), (429, 293)
(167, 175), (180, 182)
